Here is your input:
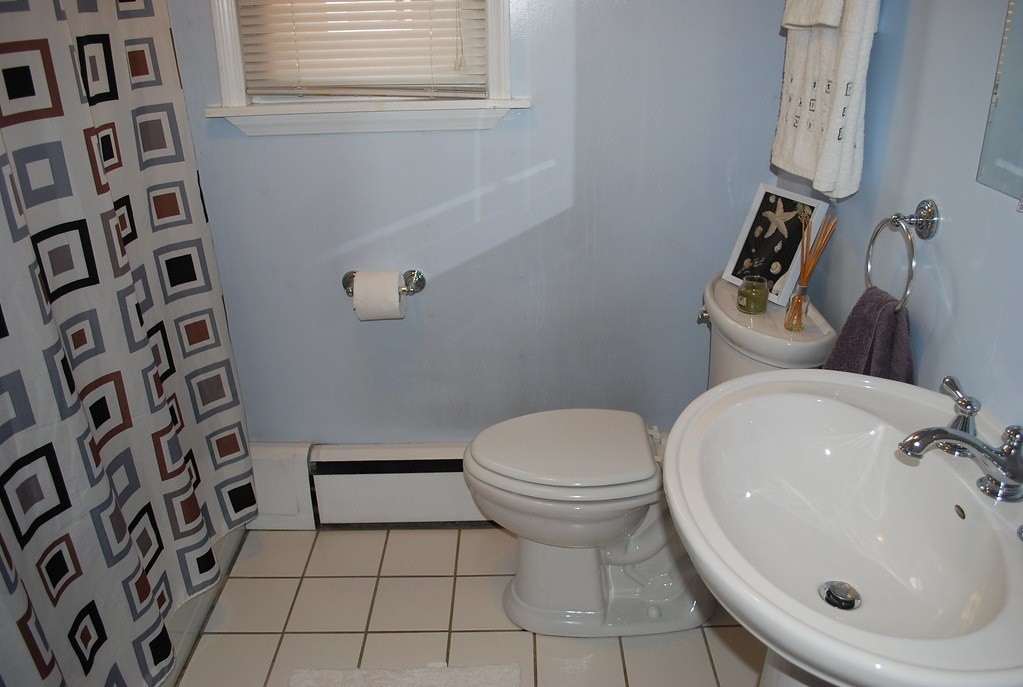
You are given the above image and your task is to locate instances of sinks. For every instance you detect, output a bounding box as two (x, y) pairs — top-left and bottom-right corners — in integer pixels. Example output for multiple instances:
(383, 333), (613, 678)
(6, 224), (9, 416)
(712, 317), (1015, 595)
(662, 368), (1022, 687)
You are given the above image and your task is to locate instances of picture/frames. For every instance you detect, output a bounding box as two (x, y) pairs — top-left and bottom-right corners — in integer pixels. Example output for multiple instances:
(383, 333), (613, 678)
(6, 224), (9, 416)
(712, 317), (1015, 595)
(721, 182), (829, 305)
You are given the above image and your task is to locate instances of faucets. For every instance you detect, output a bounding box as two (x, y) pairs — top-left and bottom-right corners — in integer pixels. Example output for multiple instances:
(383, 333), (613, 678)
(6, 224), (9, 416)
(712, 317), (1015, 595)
(896, 425), (1023, 503)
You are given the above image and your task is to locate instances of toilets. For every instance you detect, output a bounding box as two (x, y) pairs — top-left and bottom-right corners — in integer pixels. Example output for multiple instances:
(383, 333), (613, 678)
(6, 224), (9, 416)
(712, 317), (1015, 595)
(462, 267), (839, 640)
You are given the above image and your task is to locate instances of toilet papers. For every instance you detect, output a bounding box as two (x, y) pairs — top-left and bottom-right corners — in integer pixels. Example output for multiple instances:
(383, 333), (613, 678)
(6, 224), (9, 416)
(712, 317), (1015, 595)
(353, 271), (405, 321)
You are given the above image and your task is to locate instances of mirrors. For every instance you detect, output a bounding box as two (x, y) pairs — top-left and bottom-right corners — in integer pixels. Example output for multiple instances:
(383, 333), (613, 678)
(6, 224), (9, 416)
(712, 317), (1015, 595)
(975, 0), (1023, 200)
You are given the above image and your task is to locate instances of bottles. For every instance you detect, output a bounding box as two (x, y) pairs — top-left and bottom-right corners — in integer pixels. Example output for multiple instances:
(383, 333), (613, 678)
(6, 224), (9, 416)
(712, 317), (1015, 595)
(737, 274), (768, 315)
(784, 283), (810, 331)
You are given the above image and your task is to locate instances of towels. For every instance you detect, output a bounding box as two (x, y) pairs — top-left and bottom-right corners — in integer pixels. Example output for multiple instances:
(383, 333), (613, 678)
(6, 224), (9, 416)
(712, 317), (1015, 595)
(821, 284), (916, 386)
(768, 1), (880, 205)
